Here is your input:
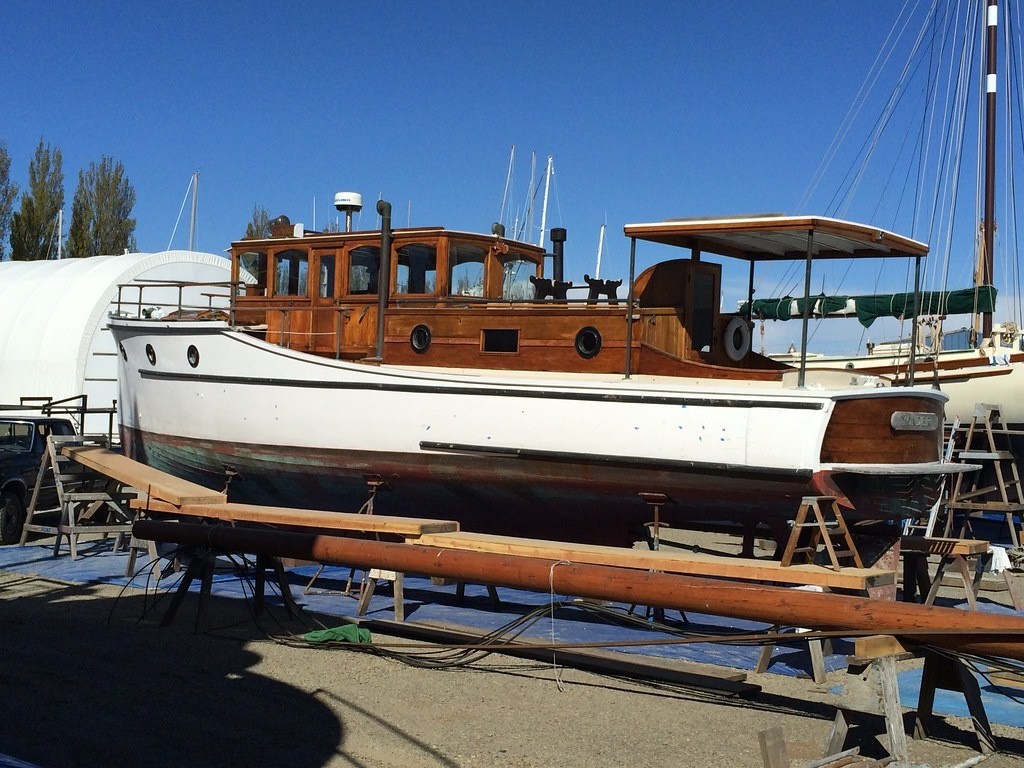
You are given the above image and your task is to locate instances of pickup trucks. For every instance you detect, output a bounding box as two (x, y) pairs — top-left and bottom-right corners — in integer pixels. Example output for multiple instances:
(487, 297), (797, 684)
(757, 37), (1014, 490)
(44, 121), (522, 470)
(0, 414), (122, 546)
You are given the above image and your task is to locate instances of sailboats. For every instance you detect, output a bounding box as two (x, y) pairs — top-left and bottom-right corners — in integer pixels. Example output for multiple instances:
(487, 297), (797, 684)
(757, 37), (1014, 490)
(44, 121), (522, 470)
(749, 0), (1023, 432)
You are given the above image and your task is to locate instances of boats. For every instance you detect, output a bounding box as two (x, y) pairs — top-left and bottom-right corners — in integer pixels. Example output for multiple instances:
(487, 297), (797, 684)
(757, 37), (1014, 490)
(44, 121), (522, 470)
(101, 188), (951, 549)
